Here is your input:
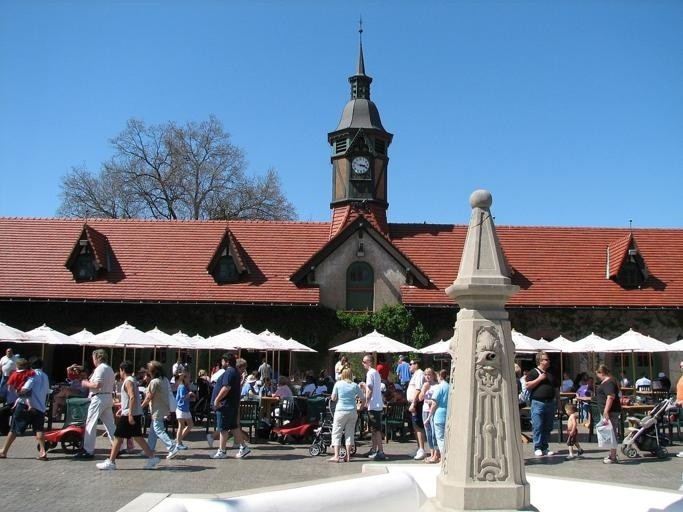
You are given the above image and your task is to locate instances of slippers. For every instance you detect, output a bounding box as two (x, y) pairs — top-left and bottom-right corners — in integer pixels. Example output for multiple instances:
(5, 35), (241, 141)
(36, 455), (48, 461)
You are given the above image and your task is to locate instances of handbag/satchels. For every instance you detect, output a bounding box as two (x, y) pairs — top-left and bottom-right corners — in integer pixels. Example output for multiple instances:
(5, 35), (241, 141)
(168, 389), (178, 413)
(595, 418), (618, 449)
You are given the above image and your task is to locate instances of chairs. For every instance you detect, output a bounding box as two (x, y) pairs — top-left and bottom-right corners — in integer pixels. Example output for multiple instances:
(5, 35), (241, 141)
(29, 387), (61, 431)
(239, 396), (308, 444)
(380, 401), (404, 443)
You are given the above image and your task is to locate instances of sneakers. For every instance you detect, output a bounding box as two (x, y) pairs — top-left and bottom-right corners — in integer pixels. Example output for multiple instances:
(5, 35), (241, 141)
(533, 449), (555, 457)
(414, 448), (427, 460)
(363, 448), (387, 460)
(235, 443), (251, 458)
(425, 456), (439, 463)
(72, 452), (94, 460)
(96, 457), (117, 469)
(176, 442), (187, 450)
(603, 455), (620, 465)
(144, 455), (161, 469)
(207, 433), (214, 447)
(166, 446), (180, 460)
(208, 449), (228, 459)
(565, 448), (584, 461)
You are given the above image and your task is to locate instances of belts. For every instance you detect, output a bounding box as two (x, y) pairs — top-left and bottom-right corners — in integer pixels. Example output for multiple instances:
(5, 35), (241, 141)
(91, 391), (112, 397)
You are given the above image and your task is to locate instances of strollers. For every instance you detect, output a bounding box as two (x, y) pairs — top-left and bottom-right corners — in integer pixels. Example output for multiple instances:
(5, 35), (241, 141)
(619, 398), (674, 459)
(34, 398), (90, 455)
(267, 396), (326, 445)
(308, 411), (358, 460)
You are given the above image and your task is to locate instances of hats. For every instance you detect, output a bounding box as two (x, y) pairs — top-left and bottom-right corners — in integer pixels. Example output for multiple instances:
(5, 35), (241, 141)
(658, 372), (665, 378)
(246, 374), (256, 384)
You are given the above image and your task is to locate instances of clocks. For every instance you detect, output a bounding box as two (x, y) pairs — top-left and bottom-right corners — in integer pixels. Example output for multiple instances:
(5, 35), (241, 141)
(351, 156), (370, 175)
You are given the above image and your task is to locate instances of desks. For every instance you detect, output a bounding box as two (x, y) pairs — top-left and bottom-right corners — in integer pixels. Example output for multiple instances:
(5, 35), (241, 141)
(560, 387), (683, 442)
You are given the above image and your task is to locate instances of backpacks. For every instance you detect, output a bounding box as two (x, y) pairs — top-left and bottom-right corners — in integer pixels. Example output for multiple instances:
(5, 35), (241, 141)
(518, 367), (542, 404)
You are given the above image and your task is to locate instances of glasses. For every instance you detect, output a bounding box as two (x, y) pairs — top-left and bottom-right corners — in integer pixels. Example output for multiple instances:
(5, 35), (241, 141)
(410, 363), (414, 366)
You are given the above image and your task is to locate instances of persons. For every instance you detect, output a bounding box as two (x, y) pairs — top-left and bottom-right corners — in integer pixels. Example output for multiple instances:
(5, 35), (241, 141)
(1, 347), (682, 471)
